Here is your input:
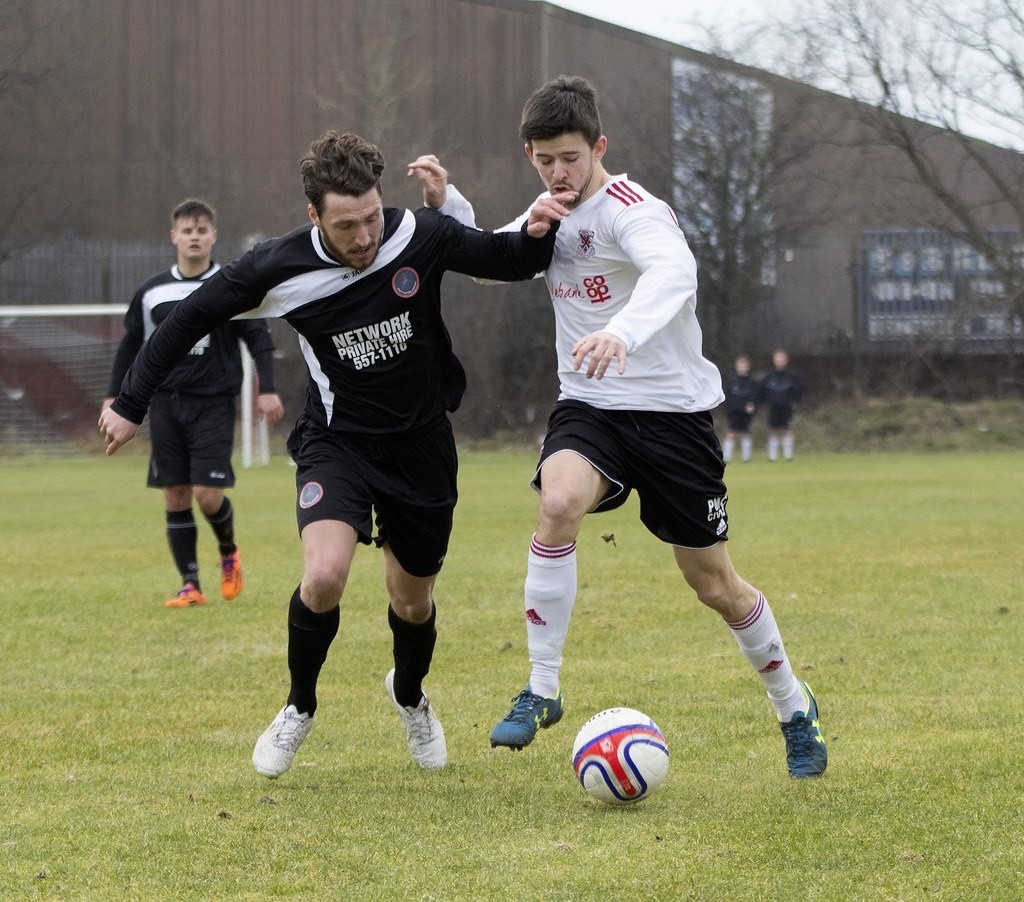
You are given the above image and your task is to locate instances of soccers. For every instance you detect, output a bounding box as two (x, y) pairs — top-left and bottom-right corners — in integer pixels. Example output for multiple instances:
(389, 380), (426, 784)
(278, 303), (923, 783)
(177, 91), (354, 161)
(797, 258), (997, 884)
(572, 707), (671, 806)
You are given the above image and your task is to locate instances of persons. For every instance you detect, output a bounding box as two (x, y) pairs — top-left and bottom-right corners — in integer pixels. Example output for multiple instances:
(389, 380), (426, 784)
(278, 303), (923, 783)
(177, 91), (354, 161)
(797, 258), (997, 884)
(762, 344), (807, 464)
(404, 74), (832, 780)
(97, 200), (285, 608)
(722, 354), (763, 464)
(98, 127), (580, 784)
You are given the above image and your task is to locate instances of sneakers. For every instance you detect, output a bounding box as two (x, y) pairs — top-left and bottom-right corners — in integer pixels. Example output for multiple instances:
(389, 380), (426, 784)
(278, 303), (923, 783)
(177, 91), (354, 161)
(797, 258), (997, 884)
(489, 682), (562, 752)
(384, 668), (447, 770)
(776, 679), (827, 780)
(164, 583), (205, 607)
(252, 702), (317, 779)
(220, 545), (242, 601)
